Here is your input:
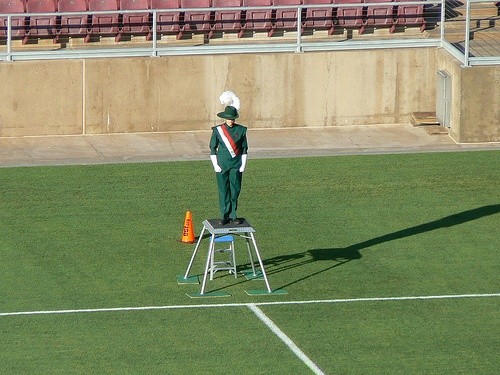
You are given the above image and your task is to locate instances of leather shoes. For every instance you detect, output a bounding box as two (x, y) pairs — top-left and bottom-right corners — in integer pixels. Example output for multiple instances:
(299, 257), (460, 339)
(231, 219), (241, 225)
(218, 220), (225, 225)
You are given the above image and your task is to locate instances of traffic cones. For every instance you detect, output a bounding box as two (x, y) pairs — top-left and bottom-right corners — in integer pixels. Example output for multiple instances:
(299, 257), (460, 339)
(176, 209), (196, 244)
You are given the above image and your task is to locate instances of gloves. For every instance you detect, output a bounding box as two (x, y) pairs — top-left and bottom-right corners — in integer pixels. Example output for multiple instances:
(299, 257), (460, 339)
(238, 153), (248, 172)
(209, 155), (223, 173)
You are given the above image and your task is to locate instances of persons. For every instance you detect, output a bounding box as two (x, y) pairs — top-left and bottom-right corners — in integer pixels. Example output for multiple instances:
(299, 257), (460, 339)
(209, 106), (248, 225)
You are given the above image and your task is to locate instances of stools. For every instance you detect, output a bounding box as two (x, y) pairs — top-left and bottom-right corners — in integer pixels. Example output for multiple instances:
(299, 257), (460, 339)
(208, 235), (238, 280)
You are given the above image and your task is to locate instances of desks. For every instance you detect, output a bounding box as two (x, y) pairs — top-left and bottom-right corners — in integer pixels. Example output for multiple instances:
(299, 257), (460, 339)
(182, 218), (272, 295)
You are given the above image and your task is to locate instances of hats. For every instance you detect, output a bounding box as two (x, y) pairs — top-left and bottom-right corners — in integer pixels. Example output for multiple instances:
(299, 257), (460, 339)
(217, 105), (239, 119)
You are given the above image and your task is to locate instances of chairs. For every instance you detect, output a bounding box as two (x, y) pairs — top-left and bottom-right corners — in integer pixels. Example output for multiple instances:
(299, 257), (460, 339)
(0, 0), (427, 46)
